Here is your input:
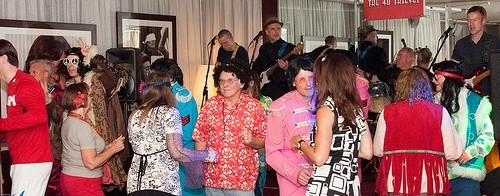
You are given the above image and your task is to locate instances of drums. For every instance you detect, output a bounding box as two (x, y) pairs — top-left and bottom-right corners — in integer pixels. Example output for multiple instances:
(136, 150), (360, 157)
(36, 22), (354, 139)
(368, 81), (393, 113)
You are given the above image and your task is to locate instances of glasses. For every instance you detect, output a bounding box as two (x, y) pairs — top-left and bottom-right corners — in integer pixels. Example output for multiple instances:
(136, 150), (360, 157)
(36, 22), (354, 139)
(63, 58), (80, 65)
(295, 76), (314, 84)
(217, 77), (238, 85)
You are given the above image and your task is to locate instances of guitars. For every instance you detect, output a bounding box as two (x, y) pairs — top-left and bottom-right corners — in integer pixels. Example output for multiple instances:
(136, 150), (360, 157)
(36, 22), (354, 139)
(259, 41), (304, 90)
(463, 66), (490, 95)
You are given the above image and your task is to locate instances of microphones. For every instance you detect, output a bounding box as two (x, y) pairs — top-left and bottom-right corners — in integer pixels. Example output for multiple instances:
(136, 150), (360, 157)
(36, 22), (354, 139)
(401, 39), (407, 47)
(249, 31), (264, 46)
(207, 35), (220, 47)
(440, 25), (454, 39)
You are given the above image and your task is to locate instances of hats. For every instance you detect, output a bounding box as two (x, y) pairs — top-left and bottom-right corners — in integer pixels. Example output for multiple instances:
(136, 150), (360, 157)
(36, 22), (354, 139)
(264, 16), (284, 28)
(359, 25), (378, 37)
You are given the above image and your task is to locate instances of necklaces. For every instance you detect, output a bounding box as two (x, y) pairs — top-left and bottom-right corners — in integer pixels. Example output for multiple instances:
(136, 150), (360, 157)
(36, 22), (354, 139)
(223, 97), (243, 135)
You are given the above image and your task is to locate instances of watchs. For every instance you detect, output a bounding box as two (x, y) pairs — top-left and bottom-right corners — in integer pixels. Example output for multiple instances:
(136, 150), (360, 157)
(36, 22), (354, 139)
(297, 140), (304, 149)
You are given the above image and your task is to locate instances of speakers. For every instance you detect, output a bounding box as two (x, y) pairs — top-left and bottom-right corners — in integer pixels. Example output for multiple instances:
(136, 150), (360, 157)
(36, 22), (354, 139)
(107, 47), (143, 103)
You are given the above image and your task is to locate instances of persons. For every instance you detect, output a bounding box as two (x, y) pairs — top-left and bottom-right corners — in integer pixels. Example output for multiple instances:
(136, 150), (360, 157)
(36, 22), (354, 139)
(0, 37), (500, 196)
(450, 6), (500, 98)
(22, 35), (71, 82)
(344, 25), (378, 76)
(250, 17), (300, 101)
(213, 28), (250, 96)
(139, 27), (169, 81)
(309, 35), (337, 63)
